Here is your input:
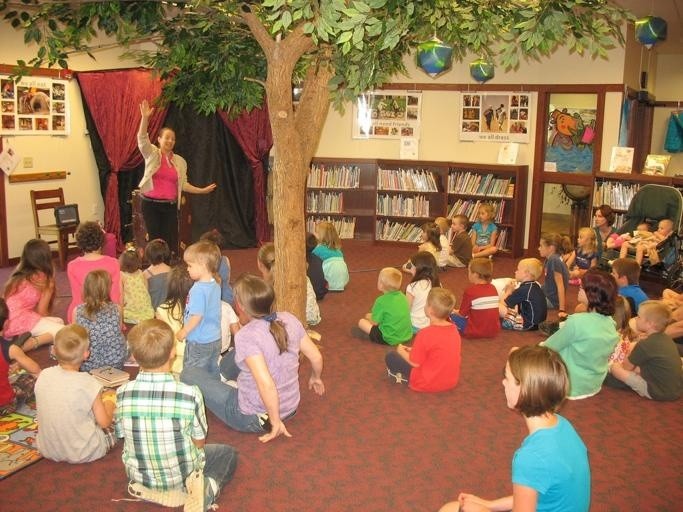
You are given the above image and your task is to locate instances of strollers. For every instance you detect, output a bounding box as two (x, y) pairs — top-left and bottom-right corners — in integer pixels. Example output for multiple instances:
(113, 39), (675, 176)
(598, 182), (682, 296)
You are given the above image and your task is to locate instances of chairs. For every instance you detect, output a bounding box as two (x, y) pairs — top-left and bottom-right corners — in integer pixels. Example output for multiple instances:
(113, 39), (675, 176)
(29, 187), (88, 273)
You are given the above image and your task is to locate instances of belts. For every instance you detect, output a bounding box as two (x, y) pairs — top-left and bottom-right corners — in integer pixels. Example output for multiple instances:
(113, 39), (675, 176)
(140, 194), (176, 203)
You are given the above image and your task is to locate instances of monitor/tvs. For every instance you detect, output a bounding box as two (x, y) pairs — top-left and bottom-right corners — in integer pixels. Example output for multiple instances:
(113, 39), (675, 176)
(54, 203), (80, 228)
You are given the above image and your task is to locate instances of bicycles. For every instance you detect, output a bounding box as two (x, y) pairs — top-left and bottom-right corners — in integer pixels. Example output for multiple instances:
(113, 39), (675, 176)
(371, 105), (406, 119)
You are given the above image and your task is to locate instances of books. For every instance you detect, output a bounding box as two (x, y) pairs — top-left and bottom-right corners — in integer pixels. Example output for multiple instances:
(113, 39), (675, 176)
(306, 162), (515, 251)
(591, 147), (682, 237)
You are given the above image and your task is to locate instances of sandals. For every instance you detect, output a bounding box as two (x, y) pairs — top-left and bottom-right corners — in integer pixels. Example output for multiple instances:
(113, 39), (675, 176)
(12, 331), (32, 348)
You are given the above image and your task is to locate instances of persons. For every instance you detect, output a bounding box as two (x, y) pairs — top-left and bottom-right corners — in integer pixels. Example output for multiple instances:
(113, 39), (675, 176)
(357, 100), (418, 136)
(358, 204), (683, 512)
(137, 100), (216, 260)
(463, 100), (529, 133)
(2, 222), (350, 512)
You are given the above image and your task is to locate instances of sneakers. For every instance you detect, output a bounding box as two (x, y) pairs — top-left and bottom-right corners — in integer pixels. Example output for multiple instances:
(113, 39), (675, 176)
(184, 469), (219, 512)
(127, 479), (187, 508)
(487, 254), (494, 260)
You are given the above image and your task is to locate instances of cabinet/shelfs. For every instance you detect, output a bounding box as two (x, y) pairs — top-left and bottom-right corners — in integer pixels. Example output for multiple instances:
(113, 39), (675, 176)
(260, 155), (528, 259)
(589, 169), (683, 235)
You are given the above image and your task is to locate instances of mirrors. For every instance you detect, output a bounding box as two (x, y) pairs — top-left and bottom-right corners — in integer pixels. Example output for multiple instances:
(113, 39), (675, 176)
(541, 91), (599, 176)
(527, 169), (593, 263)
